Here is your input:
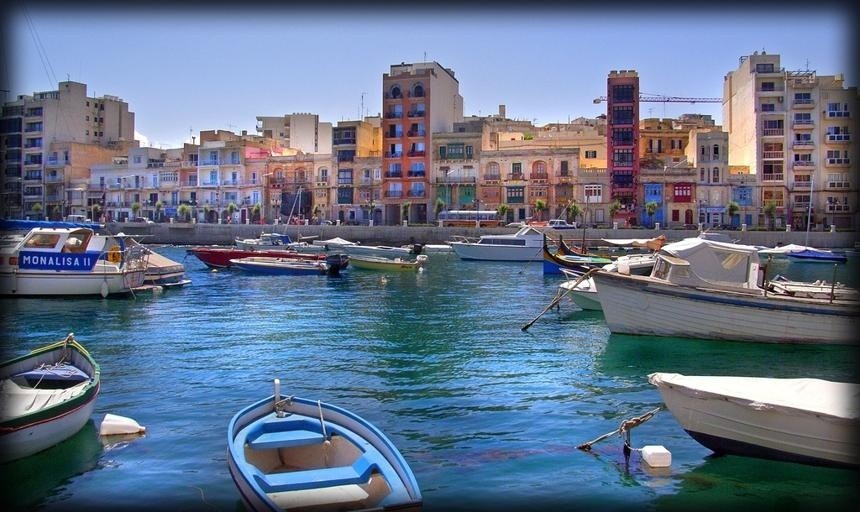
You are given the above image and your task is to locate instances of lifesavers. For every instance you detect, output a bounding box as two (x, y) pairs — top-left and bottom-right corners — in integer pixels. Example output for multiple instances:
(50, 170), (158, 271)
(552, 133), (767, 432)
(108, 246), (121, 263)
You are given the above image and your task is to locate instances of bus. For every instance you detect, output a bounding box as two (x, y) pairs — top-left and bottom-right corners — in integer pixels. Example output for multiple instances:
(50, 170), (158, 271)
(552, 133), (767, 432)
(437, 210), (505, 228)
(65, 215), (85, 222)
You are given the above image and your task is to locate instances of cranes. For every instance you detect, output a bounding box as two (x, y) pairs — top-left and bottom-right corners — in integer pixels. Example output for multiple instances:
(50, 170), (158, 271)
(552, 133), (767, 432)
(593, 92), (723, 104)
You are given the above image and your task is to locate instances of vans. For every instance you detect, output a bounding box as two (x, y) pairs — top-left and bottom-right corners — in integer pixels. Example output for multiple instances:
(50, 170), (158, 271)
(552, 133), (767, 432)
(549, 220), (567, 226)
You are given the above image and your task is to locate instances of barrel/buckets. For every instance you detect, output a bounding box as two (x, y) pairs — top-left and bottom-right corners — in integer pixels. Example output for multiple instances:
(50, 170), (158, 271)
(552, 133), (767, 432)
(642, 445), (672, 467)
(98, 413), (145, 436)
(618, 256), (631, 273)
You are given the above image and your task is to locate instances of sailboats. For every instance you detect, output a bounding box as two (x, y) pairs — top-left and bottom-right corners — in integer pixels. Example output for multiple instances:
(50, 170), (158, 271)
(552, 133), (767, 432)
(785, 173), (850, 265)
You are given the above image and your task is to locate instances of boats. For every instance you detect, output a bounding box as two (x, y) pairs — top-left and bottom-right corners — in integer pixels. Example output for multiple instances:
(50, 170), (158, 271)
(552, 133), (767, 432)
(443, 227), (553, 260)
(0, 333), (101, 460)
(228, 378), (423, 511)
(578, 252), (860, 345)
(557, 268), (603, 311)
(185, 232), (428, 275)
(647, 372), (860, 464)
(0, 227), (186, 295)
(543, 234), (834, 275)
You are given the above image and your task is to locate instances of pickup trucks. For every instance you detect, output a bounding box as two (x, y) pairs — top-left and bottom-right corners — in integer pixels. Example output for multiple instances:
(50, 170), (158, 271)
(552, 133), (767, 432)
(130, 217), (153, 224)
